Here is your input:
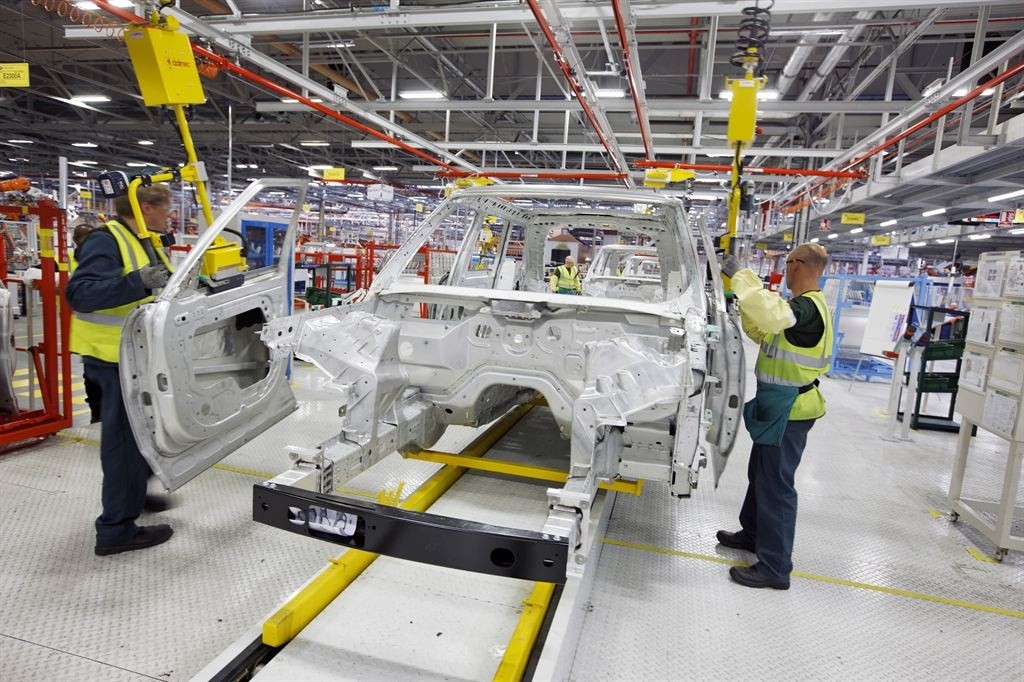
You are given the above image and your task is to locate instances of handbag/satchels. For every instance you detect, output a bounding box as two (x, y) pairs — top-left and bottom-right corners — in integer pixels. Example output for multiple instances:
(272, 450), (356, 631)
(743, 380), (798, 447)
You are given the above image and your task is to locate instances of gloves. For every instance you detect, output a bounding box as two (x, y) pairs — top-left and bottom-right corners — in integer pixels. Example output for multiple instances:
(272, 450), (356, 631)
(139, 260), (169, 289)
(720, 253), (745, 279)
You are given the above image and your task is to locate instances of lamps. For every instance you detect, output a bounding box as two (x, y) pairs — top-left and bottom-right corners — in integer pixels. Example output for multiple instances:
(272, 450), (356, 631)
(398, 89), (447, 99)
(71, 93), (112, 103)
(572, 87), (625, 97)
(921, 77), (996, 95)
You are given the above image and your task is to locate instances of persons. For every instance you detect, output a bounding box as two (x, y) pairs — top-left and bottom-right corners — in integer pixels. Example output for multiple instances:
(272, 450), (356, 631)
(716, 243), (834, 589)
(616, 264), (625, 276)
(549, 256), (581, 296)
(65, 185), (173, 554)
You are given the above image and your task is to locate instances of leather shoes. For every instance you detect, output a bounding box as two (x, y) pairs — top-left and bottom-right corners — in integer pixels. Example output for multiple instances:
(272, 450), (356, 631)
(730, 561), (790, 589)
(145, 495), (167, 510)
(95, 524), (172, 556)
(717, 529), (757, 554)
